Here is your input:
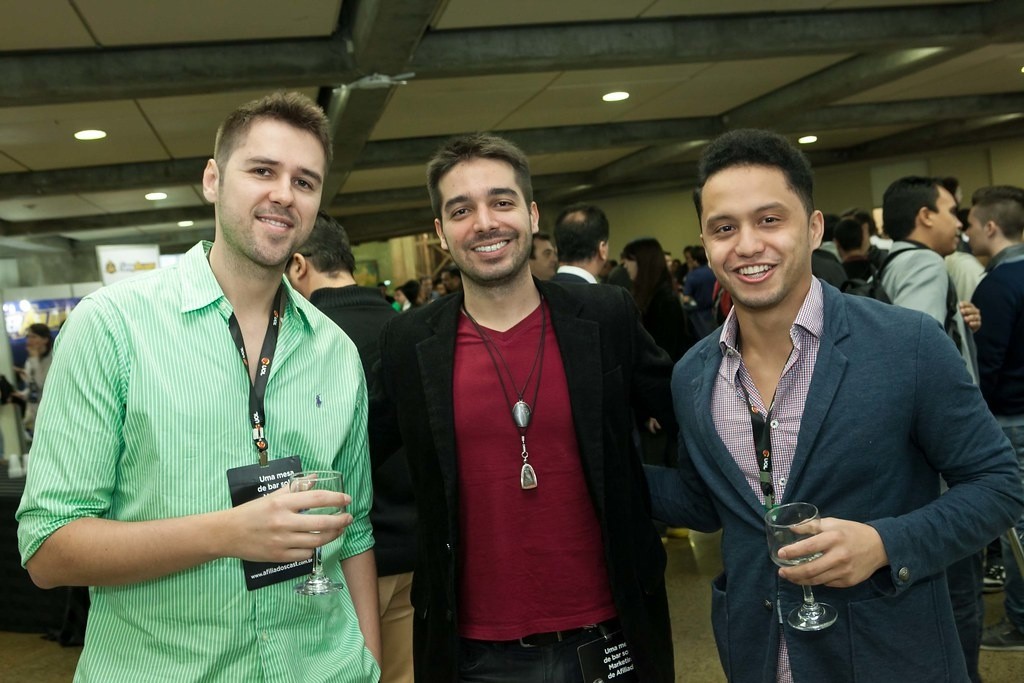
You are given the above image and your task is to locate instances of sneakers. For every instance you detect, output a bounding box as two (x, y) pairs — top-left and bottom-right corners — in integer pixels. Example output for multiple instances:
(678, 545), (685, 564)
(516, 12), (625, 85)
(981, 565), (1007, 592)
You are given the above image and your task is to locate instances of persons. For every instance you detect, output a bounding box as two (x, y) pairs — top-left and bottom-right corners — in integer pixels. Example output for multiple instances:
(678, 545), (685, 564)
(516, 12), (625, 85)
(277, 214), (469, 683)
(641, 127), (1024, 683)
(806, 174), (1024, 653)
(525, 202), (734, 537)
(371, 133), (723, 683)
(15, 89), (379, 683)
(12, 324), (56, 437)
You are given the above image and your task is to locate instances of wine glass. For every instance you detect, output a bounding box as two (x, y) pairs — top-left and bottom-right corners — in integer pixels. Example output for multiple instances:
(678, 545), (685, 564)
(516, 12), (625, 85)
(289, 470), (346, 596)
(763, 501), (839, 632)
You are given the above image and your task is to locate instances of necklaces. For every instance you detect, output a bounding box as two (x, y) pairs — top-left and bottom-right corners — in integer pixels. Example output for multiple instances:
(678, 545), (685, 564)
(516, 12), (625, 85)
(462, 300), (546, 490)
(466, 308), (543, 427)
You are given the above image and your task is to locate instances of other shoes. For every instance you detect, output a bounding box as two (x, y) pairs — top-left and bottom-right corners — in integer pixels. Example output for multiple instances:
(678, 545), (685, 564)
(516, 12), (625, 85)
(979, 616), (1024, 651)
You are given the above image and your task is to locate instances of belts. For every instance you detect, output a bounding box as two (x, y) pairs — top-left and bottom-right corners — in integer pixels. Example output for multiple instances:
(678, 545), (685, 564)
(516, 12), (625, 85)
(508, 621), (616, 648)
